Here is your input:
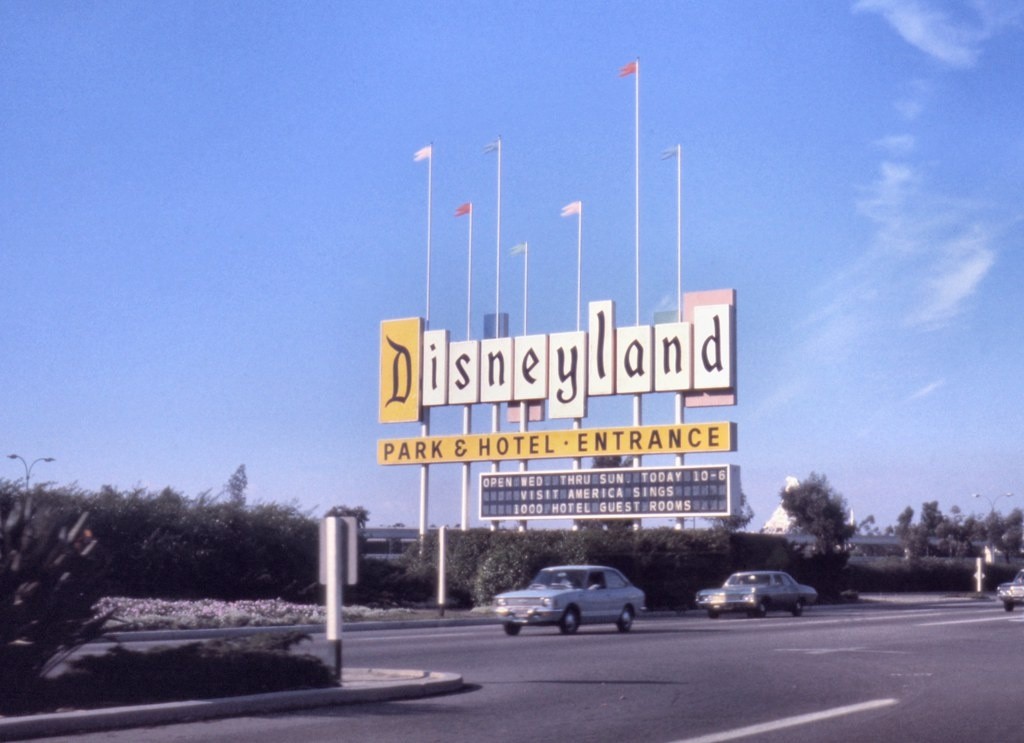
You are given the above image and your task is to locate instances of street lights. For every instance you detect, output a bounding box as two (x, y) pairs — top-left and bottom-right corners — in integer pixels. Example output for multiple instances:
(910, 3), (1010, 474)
(971, 491), (1014, 518)
(7, 457), (54, 489)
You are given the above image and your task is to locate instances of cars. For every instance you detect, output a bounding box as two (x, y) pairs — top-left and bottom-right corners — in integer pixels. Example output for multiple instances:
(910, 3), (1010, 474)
(493, 565), (647, 634)
(998, 570), (1024, 610)
(696, 572), (815, 619)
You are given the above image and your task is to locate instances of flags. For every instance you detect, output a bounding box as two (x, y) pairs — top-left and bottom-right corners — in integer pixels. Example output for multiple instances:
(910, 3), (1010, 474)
(510, 243), (526, 255)
(484, 141), (498, 153)
(455, 203), (470, 217)
(562, 201), (580, 217)
(618, 62), (637, 78)
(414, 146), (431, 161)
(661, 146), (678, 160)
